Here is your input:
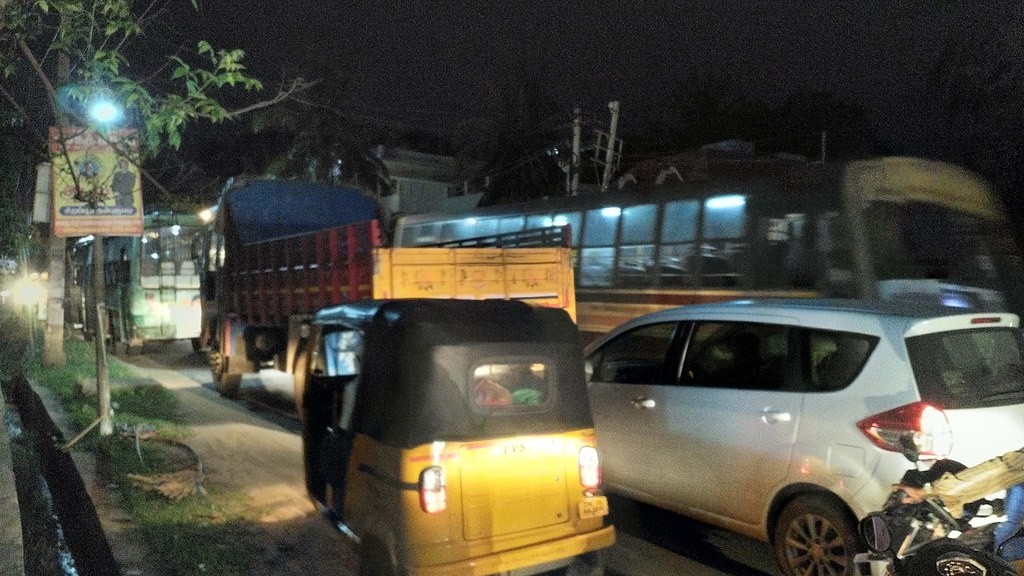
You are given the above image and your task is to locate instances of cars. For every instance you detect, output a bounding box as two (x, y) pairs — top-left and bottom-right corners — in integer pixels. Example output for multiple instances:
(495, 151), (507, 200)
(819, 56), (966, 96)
(574, 296), (1023, 576)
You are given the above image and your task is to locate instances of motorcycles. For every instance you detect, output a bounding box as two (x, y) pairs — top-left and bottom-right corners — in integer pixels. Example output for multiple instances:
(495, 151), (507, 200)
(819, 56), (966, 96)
(292, 296), (620, 576)
(862, 433), (1024, 576)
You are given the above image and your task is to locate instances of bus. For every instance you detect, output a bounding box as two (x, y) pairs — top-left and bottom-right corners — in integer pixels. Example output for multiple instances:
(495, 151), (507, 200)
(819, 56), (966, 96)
(393, 155), (1024, 325)
(60, 209), (225, 358)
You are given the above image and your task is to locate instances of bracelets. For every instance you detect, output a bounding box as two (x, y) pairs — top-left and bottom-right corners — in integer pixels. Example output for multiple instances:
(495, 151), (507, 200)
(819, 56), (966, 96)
(923, 483), (932, 498)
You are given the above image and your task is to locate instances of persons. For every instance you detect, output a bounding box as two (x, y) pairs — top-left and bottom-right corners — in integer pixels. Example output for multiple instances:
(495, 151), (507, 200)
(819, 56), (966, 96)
(81, 159), (137, 207)
(891, 446), (1024, 508)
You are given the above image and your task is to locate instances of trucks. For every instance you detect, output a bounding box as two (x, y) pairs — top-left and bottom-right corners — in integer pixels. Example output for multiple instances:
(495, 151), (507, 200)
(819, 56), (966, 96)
(193, 173), (579, 399)
